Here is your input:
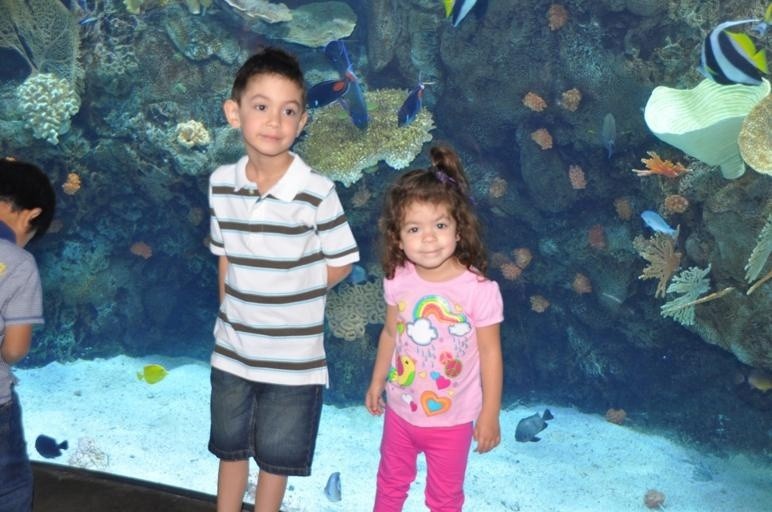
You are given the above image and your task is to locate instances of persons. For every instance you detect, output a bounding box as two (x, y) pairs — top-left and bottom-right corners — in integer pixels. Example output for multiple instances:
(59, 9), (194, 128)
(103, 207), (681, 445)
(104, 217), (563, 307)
(206, 47), (361, 511)
(363, 146), (515, 511)
(0, 159), (57, 511)
